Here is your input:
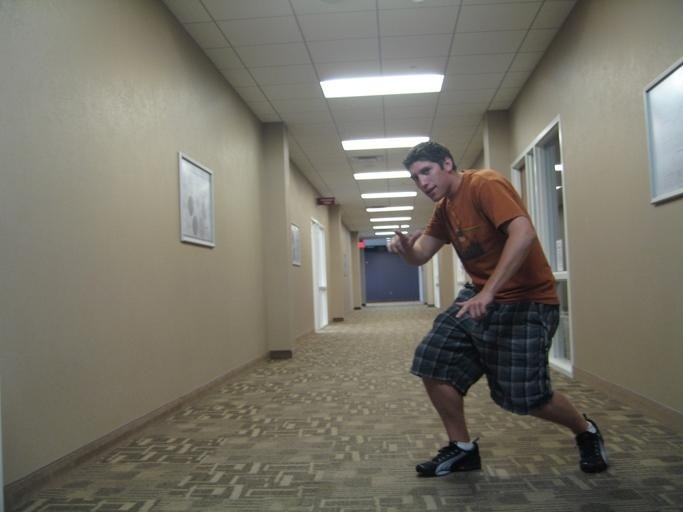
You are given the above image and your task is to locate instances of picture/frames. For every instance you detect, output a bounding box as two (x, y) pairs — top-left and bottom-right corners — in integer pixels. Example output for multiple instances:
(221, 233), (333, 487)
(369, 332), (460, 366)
(177, 151), (216, 249)
(642, 57), (683, 206)
(290, 222), (302, 267)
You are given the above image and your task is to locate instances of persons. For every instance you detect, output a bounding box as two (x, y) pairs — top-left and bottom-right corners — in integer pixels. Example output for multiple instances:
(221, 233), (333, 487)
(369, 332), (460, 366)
(384, 141), (608, 475)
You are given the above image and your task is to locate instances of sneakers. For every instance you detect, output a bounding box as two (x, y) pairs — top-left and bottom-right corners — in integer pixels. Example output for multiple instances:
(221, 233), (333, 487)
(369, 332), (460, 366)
(416, 441), (481, 476)
(575, 413), (608, 472)
